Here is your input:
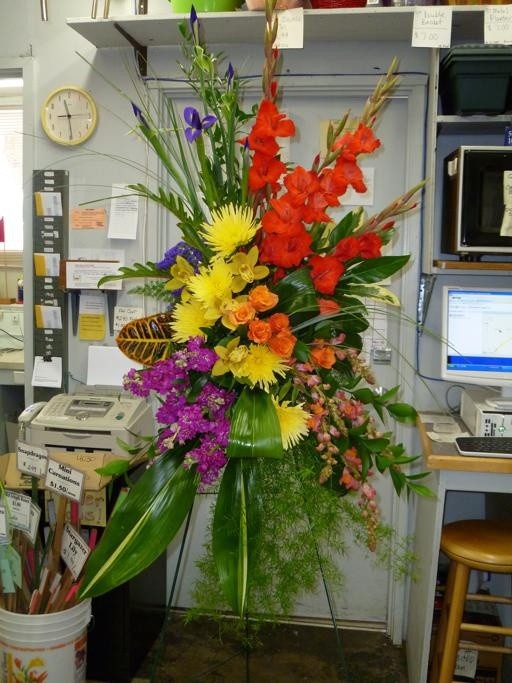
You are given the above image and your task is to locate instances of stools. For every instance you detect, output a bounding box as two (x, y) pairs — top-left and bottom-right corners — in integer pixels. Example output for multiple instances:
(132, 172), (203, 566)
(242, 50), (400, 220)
(429, 520), (512, 683)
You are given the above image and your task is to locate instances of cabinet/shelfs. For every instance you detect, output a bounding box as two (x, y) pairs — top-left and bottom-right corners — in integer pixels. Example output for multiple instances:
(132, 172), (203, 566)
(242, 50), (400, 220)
(68, 0), (421, 76)
(421, 0), (511, 277)
(1, 450), (168, 683)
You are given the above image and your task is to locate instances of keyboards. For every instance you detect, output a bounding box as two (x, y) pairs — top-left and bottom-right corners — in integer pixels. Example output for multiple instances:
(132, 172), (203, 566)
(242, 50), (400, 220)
(455, 437), (512, 459)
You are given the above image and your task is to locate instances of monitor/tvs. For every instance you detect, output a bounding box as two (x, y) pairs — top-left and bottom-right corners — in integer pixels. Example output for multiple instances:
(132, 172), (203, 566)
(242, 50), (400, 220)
(439, 285), (512, 410)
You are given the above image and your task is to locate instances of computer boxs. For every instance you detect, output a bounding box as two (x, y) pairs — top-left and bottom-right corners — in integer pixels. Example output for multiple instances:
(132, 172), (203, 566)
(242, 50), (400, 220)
(460, 387), (512, 438)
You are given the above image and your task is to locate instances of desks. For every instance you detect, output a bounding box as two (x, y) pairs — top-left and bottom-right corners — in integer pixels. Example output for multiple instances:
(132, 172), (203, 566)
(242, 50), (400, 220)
(405, 411), (512, 683)
(0, 301), (24, 451)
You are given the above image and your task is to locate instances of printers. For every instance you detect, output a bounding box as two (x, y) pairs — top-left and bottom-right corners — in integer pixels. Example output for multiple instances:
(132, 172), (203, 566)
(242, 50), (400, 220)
(19, 384), (155, 459)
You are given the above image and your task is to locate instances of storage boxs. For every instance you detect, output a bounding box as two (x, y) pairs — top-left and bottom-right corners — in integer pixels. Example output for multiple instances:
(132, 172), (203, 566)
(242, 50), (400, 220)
(427, 589), (507, 683)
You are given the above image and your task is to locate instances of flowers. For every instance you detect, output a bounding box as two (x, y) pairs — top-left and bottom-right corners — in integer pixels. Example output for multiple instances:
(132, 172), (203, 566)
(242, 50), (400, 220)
(15, 0), (434, 649)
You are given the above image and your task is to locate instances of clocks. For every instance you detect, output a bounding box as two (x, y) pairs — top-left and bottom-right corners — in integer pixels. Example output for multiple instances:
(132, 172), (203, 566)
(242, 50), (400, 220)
(39, 86), (97, 147)
